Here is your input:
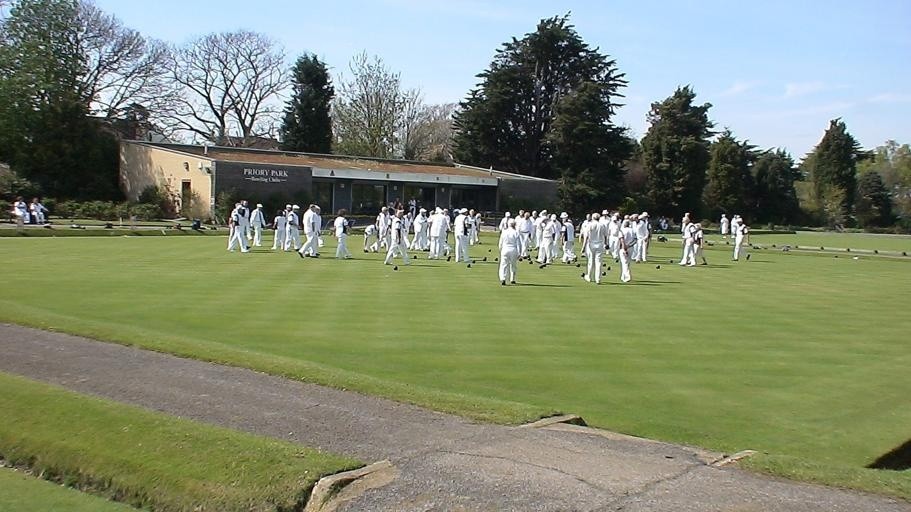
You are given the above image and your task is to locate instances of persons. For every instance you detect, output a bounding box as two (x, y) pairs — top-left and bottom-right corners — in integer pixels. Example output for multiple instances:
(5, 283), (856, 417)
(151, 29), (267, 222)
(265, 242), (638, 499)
(29, 198), (49, 225)
(14, 195), (31, 226)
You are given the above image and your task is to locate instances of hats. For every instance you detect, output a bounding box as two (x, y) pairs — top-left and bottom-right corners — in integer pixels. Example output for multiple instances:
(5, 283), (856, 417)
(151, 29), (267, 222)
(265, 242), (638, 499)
(586, 210), (650, 227)
(232, 200), (264, 222)
(505, 210), (570, 226)
(277, 204), (321, 217)
(722, 213), (744, 227)
(380, 202), (481, 217)
(682, 213), (703, 232)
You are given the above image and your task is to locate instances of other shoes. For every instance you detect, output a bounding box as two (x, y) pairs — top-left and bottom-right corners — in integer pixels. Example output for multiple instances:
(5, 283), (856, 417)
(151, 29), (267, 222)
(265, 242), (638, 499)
(363, 241), (483, 266)
(677, 254), (708, 268)
(226, 235), (263, 254)
(271, 243), (321, 258)
(336, 256), (341, 260)
(345, 255), (352, 260)
(731, 258), (739, 262)
(499, 245), (648, 286)
(746, 254), (751, 261)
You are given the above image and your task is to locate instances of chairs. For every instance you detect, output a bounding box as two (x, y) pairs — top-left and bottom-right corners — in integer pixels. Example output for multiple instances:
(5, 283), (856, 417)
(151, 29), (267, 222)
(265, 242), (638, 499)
(8, 202), (27, 224)
(28, 203), (49, 224)
